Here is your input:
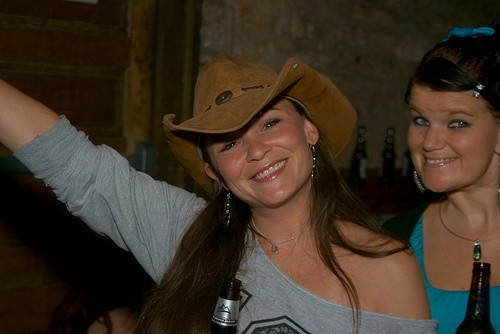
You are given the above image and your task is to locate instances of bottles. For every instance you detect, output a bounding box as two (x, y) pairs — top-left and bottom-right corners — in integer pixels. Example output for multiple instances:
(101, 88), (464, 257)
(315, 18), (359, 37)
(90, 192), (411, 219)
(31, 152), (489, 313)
(455, 262), (495, 334)
(379, 127), (395, 184)
(209, 277), (241, 334)
(400, 151), (414, 185)
(352, 127), (368, 185)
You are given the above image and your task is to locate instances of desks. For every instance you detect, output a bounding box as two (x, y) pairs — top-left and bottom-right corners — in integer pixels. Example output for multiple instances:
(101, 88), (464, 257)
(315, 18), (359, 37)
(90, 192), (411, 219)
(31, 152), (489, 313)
(340, 168), (441, 213)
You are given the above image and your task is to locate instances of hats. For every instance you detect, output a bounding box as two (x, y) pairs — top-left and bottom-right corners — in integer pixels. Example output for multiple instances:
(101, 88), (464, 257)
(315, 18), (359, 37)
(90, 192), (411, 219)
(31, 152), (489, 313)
(163, 54), (358, 198)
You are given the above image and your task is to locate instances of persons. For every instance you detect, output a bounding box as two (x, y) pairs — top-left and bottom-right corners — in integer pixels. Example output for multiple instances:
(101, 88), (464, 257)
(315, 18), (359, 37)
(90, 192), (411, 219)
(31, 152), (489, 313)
(380, 26), (500, 334)
(0, 55), (438, 334)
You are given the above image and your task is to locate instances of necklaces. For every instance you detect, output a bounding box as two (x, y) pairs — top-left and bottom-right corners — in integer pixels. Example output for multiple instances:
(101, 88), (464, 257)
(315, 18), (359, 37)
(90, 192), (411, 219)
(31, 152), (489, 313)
(439, 202), (500, 261)
(252, 218), (297, 253)
